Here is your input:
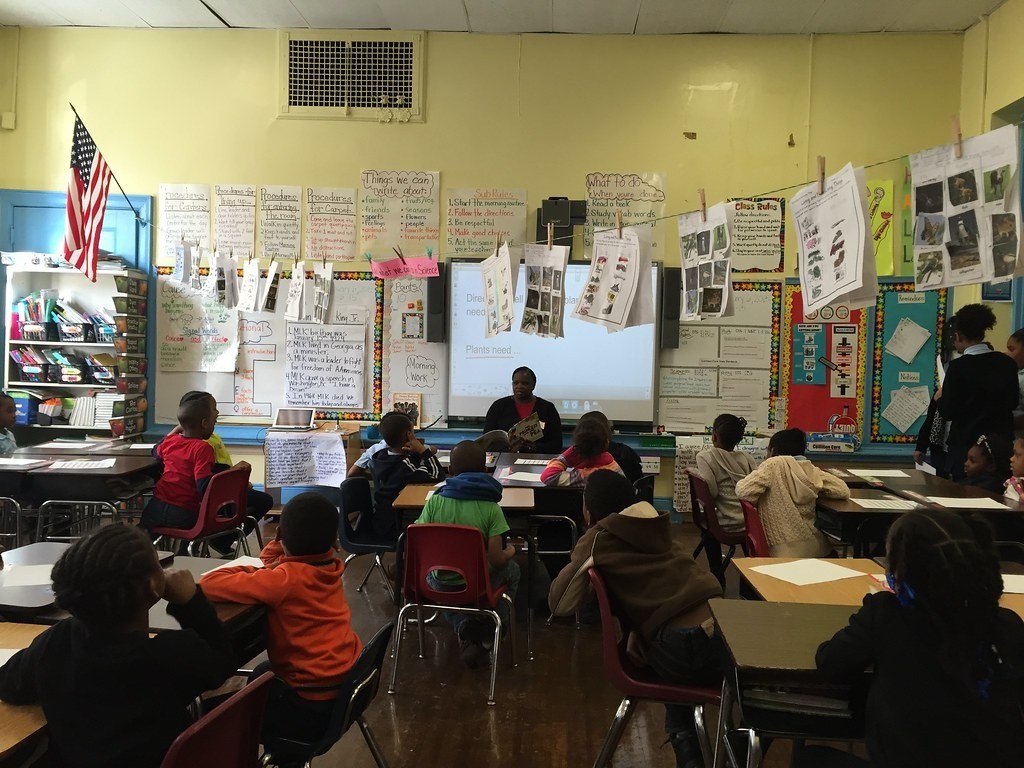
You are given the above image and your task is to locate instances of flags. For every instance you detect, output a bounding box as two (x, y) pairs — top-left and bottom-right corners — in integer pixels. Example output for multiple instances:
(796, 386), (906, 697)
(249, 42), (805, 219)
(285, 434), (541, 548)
(62, 114), (111, 283)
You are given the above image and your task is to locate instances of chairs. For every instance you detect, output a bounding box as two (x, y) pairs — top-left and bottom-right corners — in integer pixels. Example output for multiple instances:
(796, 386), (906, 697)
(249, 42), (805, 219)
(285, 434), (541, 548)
(687, 473), (744, 580)
(264, 620), (398, 768)
(387, 524), (516, 706)
(739, 500), (771, 557)
(590, 566), (722, 768)
(153, 466), (253, 559)
(155, 671), (275, 768)
(339, 477), (400, 604)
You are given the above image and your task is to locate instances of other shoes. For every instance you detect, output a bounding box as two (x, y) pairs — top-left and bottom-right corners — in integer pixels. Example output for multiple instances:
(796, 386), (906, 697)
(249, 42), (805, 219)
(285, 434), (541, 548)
(658, 728), (704, 768)
(458, 619), (507, 670)
(386, 562), (405, 587)
(728, 717), (775, 768)
(209, 544), (235, 556)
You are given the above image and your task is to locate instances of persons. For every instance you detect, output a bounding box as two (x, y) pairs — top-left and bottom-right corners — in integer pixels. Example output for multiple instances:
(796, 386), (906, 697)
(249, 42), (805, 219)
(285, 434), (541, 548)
(0, 304), (1024, 768)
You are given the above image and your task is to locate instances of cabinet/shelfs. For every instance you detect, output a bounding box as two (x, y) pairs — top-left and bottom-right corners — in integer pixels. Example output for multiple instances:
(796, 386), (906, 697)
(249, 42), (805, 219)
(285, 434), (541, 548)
(4, 268), (144, 443)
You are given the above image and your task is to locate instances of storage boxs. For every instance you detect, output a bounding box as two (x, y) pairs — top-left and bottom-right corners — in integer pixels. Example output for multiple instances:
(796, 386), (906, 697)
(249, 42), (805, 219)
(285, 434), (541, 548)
(7, 314), (118, 427)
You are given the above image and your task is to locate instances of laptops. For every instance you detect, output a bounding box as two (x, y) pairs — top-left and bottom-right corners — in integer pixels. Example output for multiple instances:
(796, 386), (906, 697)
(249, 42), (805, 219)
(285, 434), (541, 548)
(266, 407), (316, 432)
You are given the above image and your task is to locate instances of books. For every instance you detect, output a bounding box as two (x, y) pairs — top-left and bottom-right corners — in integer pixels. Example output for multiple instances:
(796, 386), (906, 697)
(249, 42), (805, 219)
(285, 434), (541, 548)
(10, 247), (144, 427)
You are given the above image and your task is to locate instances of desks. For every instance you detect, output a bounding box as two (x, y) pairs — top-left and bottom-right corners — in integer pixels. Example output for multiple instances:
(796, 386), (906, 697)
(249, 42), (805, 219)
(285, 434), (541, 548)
(389, 449), (581, 660)
(0, 540), (259, 768)
(0, 442), (162, 544)
(263, 419), (359, 523)
(710, 554), (1024, 768)
(808, 463), (1024, 561)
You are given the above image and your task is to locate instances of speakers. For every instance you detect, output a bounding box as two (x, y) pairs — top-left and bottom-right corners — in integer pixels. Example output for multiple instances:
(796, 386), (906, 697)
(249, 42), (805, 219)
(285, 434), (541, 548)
(661, 267), (681, 349)
(427, 262), (445, 342)
